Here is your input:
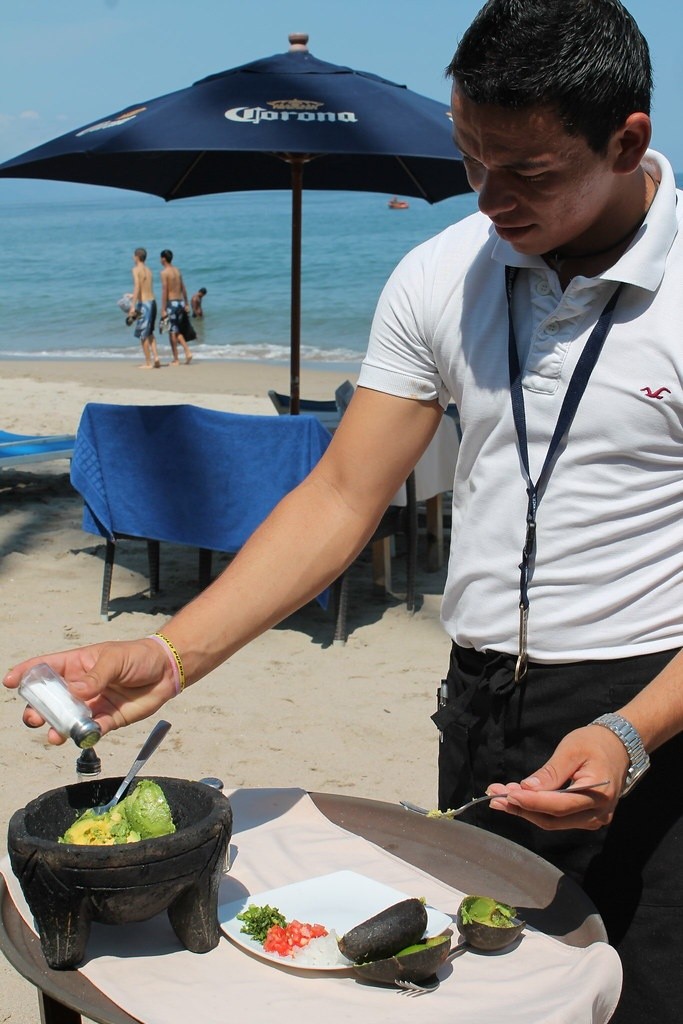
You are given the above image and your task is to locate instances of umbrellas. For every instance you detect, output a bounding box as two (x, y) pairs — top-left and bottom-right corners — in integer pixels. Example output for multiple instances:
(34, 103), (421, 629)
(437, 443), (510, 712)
(0, 33), (474, 416)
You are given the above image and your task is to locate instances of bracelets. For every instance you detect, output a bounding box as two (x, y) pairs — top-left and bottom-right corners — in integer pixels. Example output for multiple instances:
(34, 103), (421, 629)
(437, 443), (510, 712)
(145, 632), (185, 695)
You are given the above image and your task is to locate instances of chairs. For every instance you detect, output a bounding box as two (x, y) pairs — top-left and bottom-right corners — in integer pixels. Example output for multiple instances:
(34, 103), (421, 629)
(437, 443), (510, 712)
(69, 403), (407, 648)
(269, 390), (337, 414)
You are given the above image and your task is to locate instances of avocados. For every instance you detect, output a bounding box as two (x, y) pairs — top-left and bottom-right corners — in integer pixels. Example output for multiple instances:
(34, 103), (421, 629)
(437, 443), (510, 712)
(337, 897), (428, 963)
(62, 778), (176, 846)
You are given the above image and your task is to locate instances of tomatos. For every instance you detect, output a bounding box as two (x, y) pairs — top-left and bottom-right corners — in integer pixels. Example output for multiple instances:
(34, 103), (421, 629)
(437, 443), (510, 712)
(264, 920), (327, 956)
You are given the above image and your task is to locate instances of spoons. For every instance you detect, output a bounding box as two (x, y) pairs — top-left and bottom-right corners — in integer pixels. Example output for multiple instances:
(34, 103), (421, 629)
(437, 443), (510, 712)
(93, 720), (172, 815)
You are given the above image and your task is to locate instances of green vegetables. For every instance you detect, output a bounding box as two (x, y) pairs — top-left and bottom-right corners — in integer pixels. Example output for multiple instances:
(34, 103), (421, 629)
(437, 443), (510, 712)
(235, 903), (287, 944)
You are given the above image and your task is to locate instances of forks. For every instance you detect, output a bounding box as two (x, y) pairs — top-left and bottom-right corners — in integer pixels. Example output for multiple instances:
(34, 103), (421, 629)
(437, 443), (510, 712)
(400, 779), (610, 818)
(395, 940), (468, 992)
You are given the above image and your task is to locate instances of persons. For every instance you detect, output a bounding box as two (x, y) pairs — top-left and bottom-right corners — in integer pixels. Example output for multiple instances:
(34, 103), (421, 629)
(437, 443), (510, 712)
(2, 0), (683, 1024)
(159, 250), (192, 366)
(191, 287), (207, 318)
(126, 248), (160, 369)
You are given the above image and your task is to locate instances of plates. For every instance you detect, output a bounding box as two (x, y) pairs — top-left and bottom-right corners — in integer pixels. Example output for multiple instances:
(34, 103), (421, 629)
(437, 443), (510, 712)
(217, 869), (453, 970)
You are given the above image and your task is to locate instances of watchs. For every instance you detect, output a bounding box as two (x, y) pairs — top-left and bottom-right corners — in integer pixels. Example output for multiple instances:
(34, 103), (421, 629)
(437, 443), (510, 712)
(588, 713), (650, 796)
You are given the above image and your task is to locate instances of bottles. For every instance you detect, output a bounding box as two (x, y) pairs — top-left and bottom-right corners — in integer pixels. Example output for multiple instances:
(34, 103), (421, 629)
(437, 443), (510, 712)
(18, 662), (102, 749)
(75, 747), (102, 783)
(200, 776), (230, 872)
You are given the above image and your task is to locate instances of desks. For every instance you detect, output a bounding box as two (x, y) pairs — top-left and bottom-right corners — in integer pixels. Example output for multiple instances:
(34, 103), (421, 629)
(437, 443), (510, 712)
(0, 788), (624, 1024)
(299, 410), (462, 571)
